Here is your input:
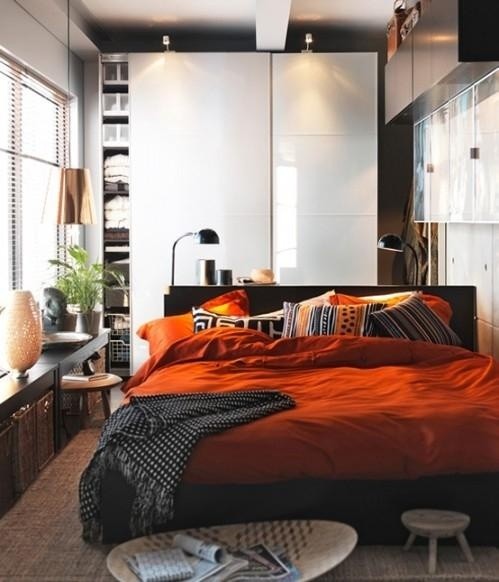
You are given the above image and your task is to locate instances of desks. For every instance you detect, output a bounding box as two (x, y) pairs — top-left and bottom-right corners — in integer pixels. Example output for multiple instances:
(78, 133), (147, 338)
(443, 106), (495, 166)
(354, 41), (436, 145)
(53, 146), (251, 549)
(0, 327), (115, 519)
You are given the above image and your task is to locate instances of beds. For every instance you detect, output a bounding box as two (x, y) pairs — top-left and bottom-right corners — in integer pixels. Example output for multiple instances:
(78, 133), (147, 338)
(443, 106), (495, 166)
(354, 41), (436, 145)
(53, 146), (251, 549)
(111, 284), (498, 547)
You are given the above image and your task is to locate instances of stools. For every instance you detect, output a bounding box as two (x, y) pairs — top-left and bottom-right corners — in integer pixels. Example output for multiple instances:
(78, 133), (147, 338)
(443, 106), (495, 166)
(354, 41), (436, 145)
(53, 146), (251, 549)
(399, 509), (475, 576)
(61, 372), (123, 429)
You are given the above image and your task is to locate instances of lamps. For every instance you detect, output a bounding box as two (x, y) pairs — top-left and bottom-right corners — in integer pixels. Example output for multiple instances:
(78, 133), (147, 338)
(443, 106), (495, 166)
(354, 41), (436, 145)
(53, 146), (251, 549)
(0, 291), (43, 380)
(376, 233), (420, 285)
(40, 165), (95, 295)
(162, 35), (175, 53)
(170, 227), (220, 285)
(301, 33), (313, 52)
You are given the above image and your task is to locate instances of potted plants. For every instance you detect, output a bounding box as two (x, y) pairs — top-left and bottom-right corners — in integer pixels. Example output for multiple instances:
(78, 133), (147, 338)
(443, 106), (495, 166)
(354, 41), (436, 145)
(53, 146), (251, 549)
(41, 242), (129, 342)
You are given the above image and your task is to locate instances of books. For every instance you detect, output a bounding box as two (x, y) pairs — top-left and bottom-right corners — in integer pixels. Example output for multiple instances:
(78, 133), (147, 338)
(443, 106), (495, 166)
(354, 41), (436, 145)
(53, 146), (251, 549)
(122, 532), (303, 581)
(234, 275), (277, 287)
(61, 372), (109, 383)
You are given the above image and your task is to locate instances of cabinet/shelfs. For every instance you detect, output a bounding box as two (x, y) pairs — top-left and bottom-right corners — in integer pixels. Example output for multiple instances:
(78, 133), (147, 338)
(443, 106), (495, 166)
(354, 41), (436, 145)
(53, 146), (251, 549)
(438, 92), (499, 365)
(384, 0), (499, 126)
